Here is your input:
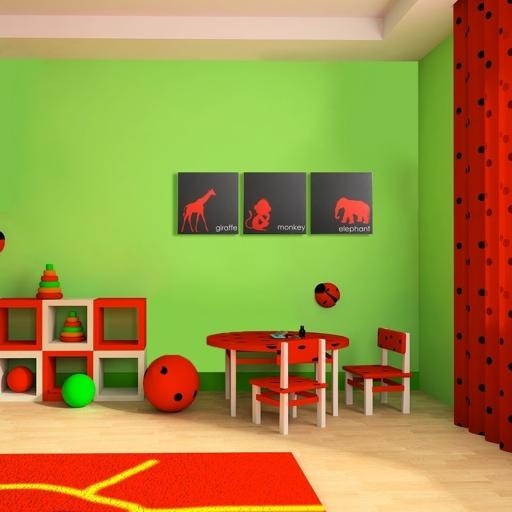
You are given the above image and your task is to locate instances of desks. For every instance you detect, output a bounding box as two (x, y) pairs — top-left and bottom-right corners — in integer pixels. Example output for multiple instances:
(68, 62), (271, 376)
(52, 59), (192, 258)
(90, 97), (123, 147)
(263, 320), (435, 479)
(203, 330), (351, 418)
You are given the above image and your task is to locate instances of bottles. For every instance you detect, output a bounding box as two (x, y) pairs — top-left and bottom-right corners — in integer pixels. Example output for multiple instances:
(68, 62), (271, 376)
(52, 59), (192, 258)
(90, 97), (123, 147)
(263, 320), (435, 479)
(299, 325), (306, 337)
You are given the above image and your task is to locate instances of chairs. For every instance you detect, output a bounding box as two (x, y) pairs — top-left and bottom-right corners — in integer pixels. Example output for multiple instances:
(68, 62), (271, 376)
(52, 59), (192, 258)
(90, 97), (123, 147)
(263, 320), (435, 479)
(340, 326), (417, 417)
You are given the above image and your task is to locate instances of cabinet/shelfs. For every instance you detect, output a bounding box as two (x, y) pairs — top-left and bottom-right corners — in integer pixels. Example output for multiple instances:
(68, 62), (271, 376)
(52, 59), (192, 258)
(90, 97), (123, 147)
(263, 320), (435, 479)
(0, 294), (149, 404)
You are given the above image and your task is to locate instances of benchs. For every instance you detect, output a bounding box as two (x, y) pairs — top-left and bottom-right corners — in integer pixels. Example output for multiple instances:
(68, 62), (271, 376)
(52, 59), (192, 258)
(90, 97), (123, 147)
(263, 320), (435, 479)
(249, 337), (328, 436)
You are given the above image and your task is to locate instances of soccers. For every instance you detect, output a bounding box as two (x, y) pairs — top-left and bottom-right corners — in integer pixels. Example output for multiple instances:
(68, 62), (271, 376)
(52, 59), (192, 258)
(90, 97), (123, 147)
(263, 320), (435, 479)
(144, 356), (200, 413)
(8, 366), (33, 392)
(61, 373), (96, 408)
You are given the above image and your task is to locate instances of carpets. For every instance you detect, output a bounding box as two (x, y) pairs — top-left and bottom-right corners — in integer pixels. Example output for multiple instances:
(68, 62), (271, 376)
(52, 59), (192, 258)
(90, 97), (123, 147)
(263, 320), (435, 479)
(1, 449), (326, 512)
